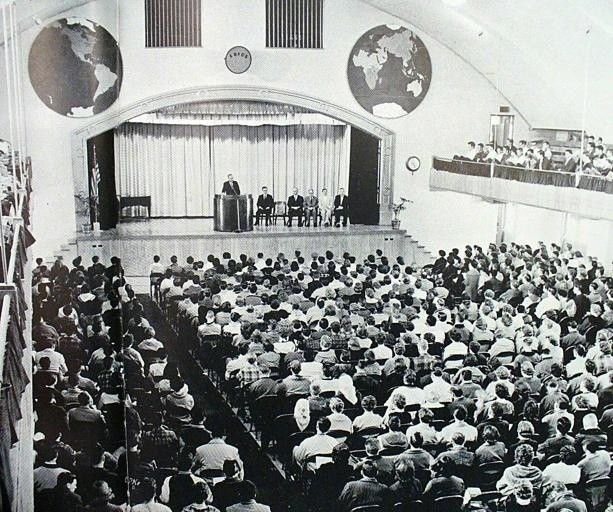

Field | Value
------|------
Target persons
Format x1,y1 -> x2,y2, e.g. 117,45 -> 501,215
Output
254,187 -> 276,225
320,187 -> 334,226
31,257 -> 268,512
146,242 -> 612,512
288,187 -> 304,227
304,190 -> 319,228
334,187 -> 350,228
221,173 -> 241,195
453,132 -> 613,188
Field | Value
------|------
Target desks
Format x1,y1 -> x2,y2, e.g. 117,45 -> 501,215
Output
121,195 -> 149,221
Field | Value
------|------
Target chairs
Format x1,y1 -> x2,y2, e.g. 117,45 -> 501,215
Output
258,200 -> 347,226
32,250 -> 613,510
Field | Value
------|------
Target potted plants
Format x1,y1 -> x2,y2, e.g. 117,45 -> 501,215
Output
387,197 -> 414,230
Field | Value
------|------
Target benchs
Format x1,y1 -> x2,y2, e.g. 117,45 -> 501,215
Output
453,139 -> 612,193
